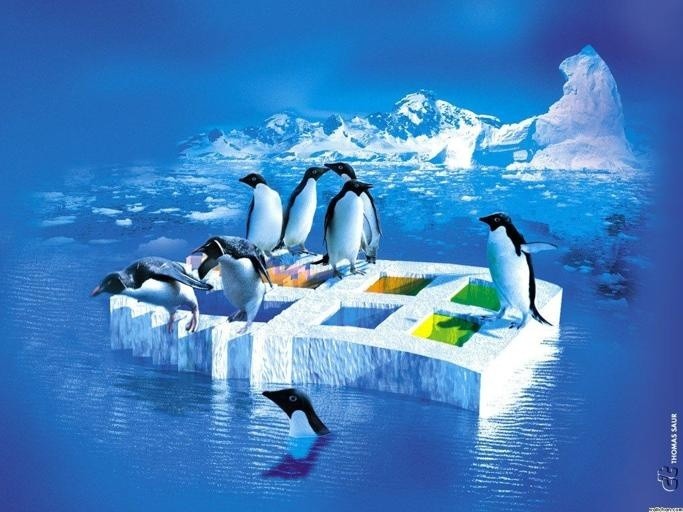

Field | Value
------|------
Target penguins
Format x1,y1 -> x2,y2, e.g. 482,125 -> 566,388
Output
89,256 -> 213,332
259,434 -> 327,481
190,235 -> 273,334
263,386 -> 332,435
323,179 -> 374,279
271,166 -> 332,256
238,172 -> 283,259
475,211 -> 559,333
324,162 -> 385,263
